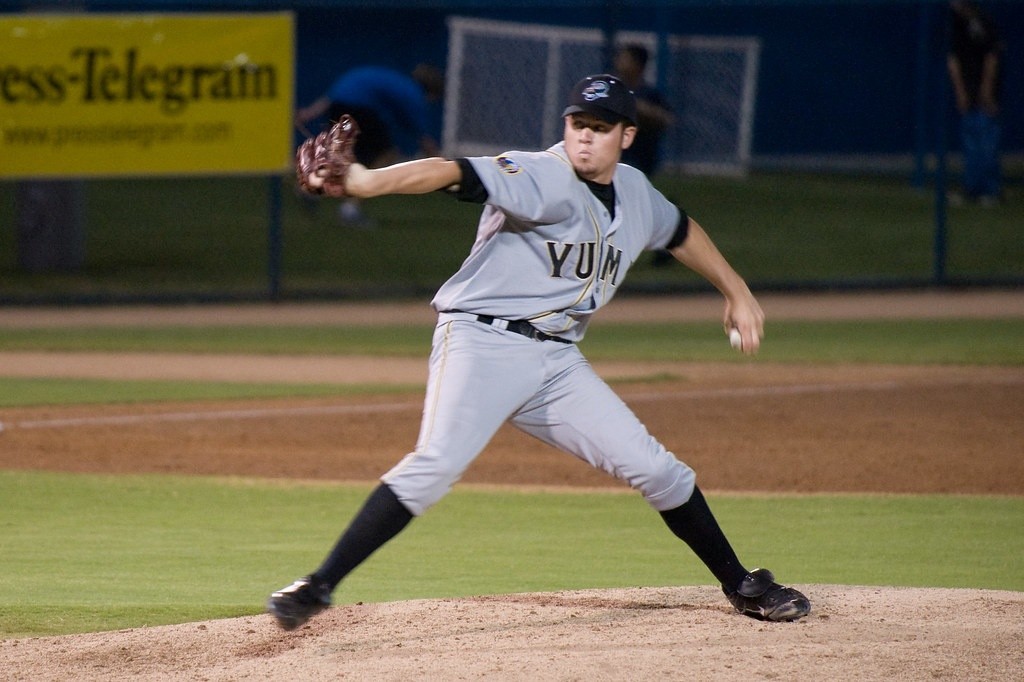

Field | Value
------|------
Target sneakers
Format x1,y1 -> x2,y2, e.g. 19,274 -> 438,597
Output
271,576 -> 331,630
721,568 -> 811,622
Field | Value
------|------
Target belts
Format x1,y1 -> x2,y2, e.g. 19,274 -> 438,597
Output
440,309 -> 572,345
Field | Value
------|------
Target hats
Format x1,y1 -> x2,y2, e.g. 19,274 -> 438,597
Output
562,74 -> 637,125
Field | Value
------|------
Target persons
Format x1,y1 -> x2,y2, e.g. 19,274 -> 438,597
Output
269,73 -> 810,630
296,60 -> 441,171
943,1 -> 1006,210
611,44 -> 675,177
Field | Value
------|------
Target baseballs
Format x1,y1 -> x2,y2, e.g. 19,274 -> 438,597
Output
729,323 -> 759,352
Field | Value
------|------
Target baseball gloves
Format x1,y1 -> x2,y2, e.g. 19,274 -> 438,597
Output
293,110 -> 360,201
